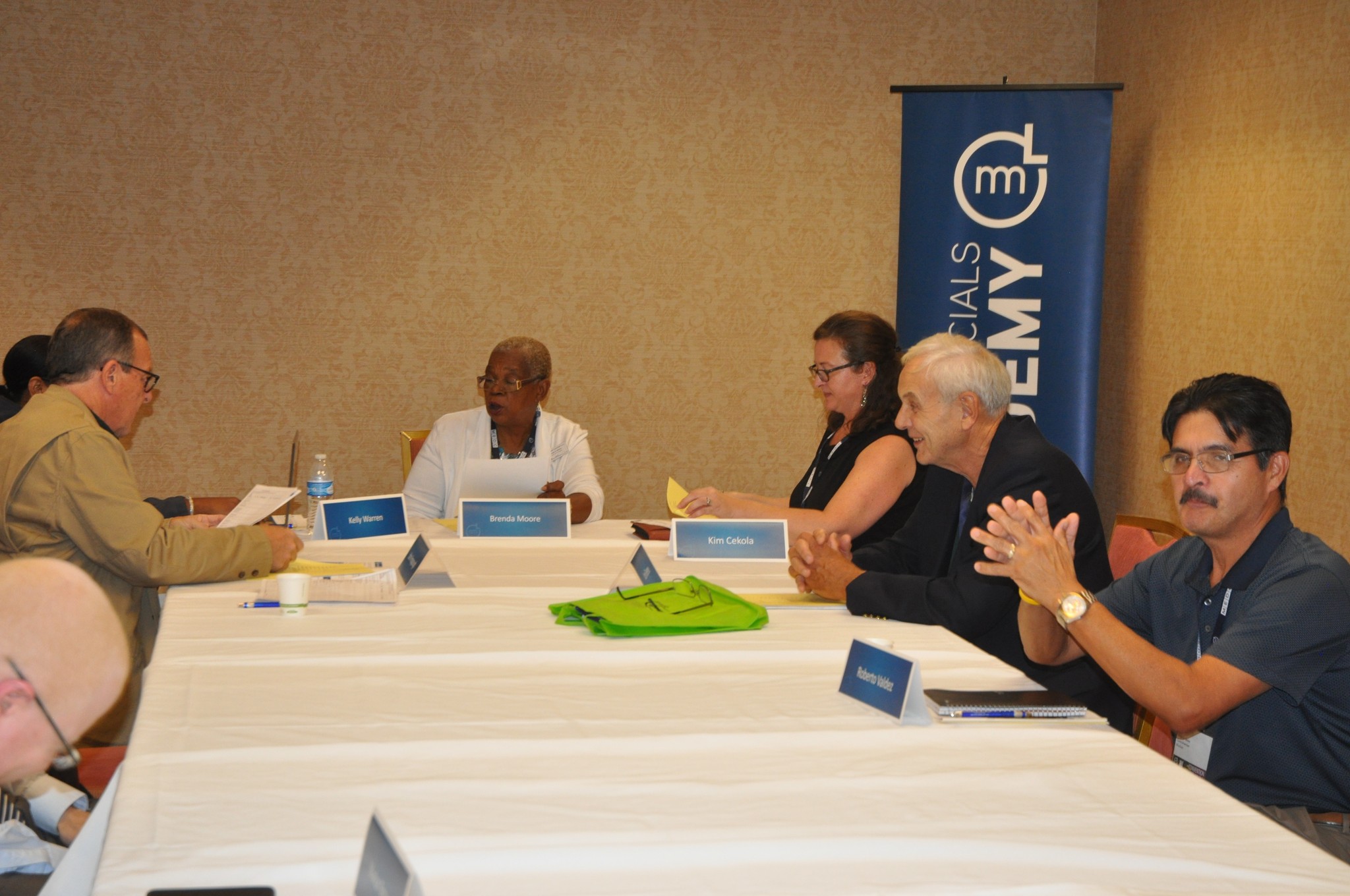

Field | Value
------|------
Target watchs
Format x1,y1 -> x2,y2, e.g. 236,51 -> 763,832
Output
1054,589 -> 1098,630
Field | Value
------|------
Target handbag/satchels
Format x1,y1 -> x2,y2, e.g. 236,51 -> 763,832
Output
548,577 -> 769,638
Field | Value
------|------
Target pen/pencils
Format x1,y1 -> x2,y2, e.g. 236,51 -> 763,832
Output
953,710 -> 1032,718
269,524 -> 296,528
238,601 -> 280,609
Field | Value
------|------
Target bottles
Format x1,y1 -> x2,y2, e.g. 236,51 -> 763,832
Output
307,453 -> 335,529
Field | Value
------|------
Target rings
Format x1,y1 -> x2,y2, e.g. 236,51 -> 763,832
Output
1007,543 -> 1016,559
721,490 -> 724,494
707,497 -> 710,505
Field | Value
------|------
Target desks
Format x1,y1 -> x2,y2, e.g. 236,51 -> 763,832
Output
88,517 -> 1350,896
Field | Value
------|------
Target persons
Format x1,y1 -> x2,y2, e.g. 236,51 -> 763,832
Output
0,307 -> 305,748
676,309 -> 938,553
787,332 -> 1134,737
0,334 -> 272,527
0,556 -> 134,896
399,336 -> 604,526
969,372 -> 1350,867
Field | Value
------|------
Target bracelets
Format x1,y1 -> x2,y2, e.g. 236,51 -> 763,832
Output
185,496 -> 194,515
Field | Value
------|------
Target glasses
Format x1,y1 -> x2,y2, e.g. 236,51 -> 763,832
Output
477,375 -> 544,391
100,360 -> 160,393
1,655 -> 80,772
808,359 -> 866,381
1157,448 -> 1269,475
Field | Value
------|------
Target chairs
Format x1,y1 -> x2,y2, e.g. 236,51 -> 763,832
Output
1103,517 -> 1200,763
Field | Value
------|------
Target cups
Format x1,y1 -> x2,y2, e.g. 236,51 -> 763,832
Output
290,529 -> 313,560
276,572 -> 310,612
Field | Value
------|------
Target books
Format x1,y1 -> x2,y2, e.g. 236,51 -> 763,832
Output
923,688 -> 1111,727
